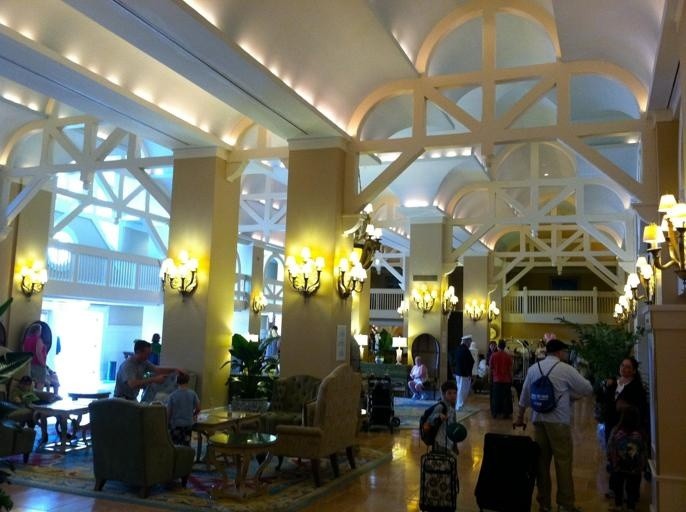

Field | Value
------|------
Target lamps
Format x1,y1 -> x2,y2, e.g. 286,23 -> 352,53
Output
160,251 -> 198,296
285,204 -> 382,300
16,260 -> 48,297
612,193 -> 686,327
397,284 -> 499,322
392,336 -> 407,366
253,291 -> 268,314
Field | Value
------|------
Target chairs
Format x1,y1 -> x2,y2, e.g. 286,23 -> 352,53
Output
412,333 -> 440,400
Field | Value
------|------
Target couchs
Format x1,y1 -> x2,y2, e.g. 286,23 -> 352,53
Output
360,363 -> 409,397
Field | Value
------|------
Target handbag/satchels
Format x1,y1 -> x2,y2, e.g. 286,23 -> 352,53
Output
607,431 -> 647,474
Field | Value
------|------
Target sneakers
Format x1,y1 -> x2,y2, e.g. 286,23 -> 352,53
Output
412,394 -> 428,400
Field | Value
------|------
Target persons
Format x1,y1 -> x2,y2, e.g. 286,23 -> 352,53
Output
148,334 -> 162,366
12,323 -> 52,415
420,382 -> 459,458
453,335 -> 548,419
166,374 -> 201,448
595,357 -> 651,512
258,326 -> 281,358
407,356 -> 428,400
114,341 -> 187,401
515,339 -> 594,512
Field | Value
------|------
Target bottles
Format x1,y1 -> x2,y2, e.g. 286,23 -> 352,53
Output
227,404 -> 232,419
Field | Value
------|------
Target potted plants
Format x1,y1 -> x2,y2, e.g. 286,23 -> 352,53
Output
375,351 -> 384,364
221,334 -> 280,430
558,318 -> 645,454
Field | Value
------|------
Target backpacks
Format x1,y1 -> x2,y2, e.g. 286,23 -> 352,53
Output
530,361 -> 562,413
420,402 -> 446,445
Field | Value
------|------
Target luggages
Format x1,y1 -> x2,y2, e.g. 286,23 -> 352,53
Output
475,423 -> 539,512
420,451 -> 459,512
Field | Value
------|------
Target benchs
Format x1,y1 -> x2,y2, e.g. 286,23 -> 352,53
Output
470,376 -> 488,394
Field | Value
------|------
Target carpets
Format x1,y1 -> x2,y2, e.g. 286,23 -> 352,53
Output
1,443 -> 392,512
394,395 -> 481,430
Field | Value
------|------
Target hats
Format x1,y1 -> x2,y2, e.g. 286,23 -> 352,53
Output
447,423 -> 466,442
546,340 -> 570,352
